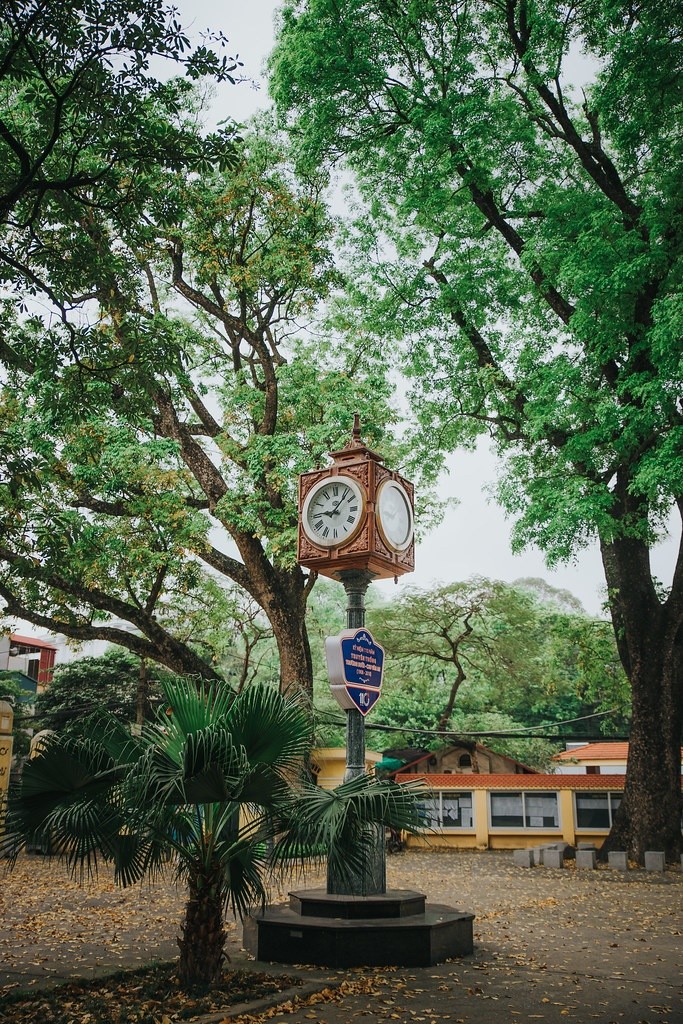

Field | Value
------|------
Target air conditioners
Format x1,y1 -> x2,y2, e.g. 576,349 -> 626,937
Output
444,769 -> 456,775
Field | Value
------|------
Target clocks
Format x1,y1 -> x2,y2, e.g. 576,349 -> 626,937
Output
303,475 -> 366,548
376,478 -> 414,552
